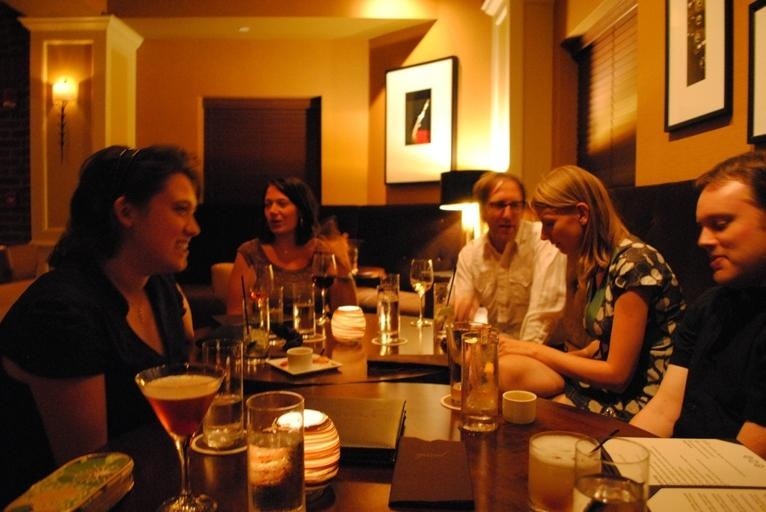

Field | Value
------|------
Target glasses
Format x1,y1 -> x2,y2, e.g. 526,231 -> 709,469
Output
486,201 -> 526,209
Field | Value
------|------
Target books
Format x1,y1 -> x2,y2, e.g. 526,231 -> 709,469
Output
303,394 -> 411,465
592,434 -> 766,512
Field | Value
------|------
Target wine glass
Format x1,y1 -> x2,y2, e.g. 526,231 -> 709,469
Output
248,263 -> 274,308
135,362 -> 227,512
409,258 -> 434,329
310,252 -> 338,327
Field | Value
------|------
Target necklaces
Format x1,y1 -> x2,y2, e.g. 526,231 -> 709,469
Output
120,287 -> 152,326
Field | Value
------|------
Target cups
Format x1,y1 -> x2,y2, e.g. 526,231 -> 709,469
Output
526,428 -> 651,512
244,390 -> 308,512
286,346 -> 314,373
200,337 -> 244,448
375,272 -> 400,344
241,297 -> 271,363
432,283 -> 456,341
268,285 -> 284,330
447,320 -> 537,434
291,281 -> 315,340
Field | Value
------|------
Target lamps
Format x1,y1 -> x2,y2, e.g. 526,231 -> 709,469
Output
52,76 -> 78,161
439,171 -> 491,234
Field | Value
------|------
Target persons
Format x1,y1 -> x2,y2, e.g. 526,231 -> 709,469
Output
446,172 -> 571,360
222,179 -> 360,316
630,152 -> 764,461
475,165 -> 690,428
0,148 -> 200,472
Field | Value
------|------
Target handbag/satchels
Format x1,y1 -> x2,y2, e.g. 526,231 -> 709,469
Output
2,454 -> 134,511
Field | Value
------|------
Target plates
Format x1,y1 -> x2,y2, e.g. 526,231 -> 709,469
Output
266,352 -> 342,376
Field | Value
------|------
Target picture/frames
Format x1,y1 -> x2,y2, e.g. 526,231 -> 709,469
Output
384,55 -> 459,187
748,1 -> 766,145
664,1 -> 733,132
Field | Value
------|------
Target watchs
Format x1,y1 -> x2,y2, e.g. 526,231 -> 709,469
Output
335,272 -> 355,284
